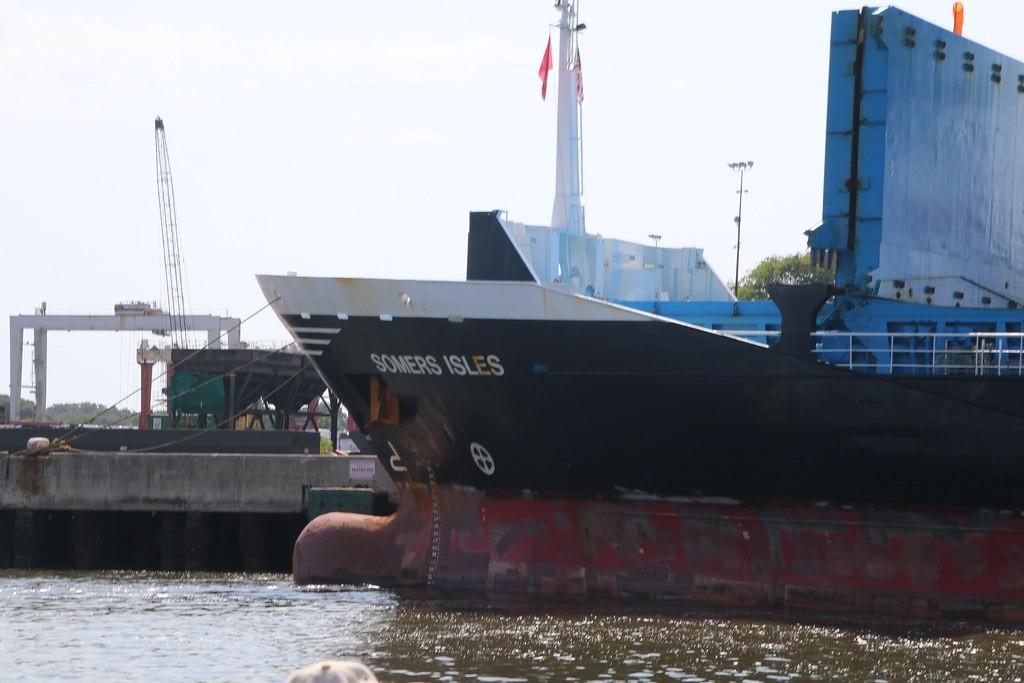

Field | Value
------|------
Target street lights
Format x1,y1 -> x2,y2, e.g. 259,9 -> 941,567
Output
727,160 -> 754,297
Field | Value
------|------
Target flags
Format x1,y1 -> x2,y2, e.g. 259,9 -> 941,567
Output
572,45 -> 584,106
537,34 -> 553,100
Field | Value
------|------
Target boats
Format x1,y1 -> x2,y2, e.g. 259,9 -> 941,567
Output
252,0 -> 1024,620
